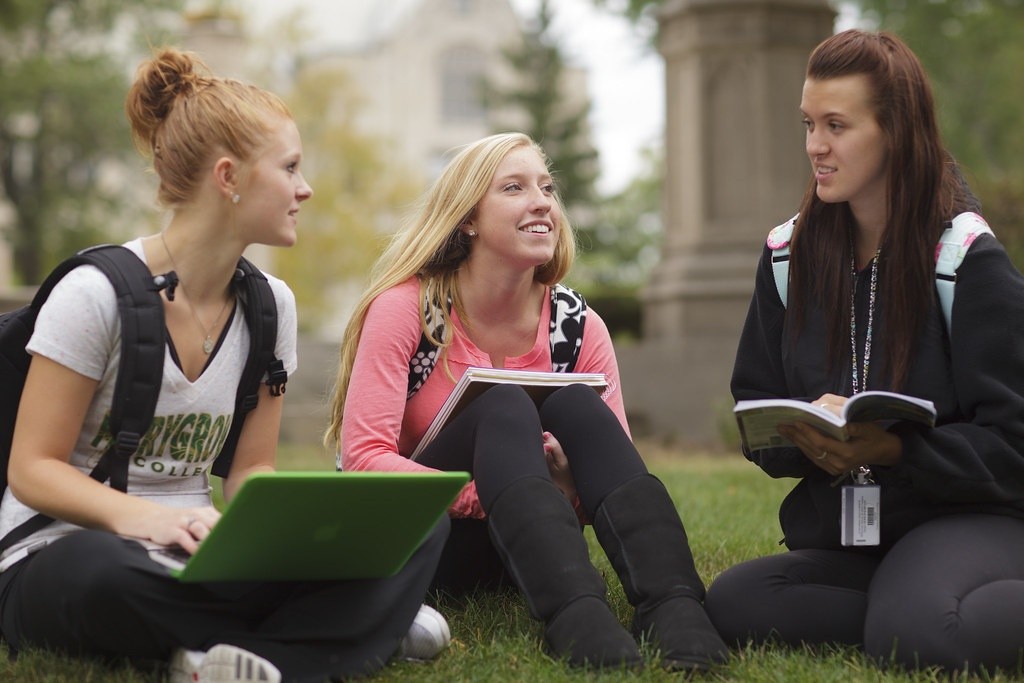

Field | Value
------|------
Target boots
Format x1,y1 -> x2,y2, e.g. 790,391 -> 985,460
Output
487,475 -> 643,671
591,472 -> 730,672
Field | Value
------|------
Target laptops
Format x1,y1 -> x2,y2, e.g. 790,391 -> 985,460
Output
115,471 -> 472,584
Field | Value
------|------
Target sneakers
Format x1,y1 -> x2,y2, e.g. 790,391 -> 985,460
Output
160,643 -> 283,683
394,604 -> 451,660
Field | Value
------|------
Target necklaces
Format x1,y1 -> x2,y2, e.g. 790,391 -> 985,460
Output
158,231 -> 231,356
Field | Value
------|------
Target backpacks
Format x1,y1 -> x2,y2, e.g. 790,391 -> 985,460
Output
0,243 -> 288,553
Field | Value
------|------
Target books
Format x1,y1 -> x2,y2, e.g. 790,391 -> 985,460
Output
733,390 -> 936,457
409,366 -> 607,467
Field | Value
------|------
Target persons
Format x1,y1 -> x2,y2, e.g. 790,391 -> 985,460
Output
706,27 -> 1024,682
326,130 -> 738,672
0,48 -> 449,682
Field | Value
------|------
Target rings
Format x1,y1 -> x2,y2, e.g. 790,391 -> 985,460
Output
817,451 -> 828,459
